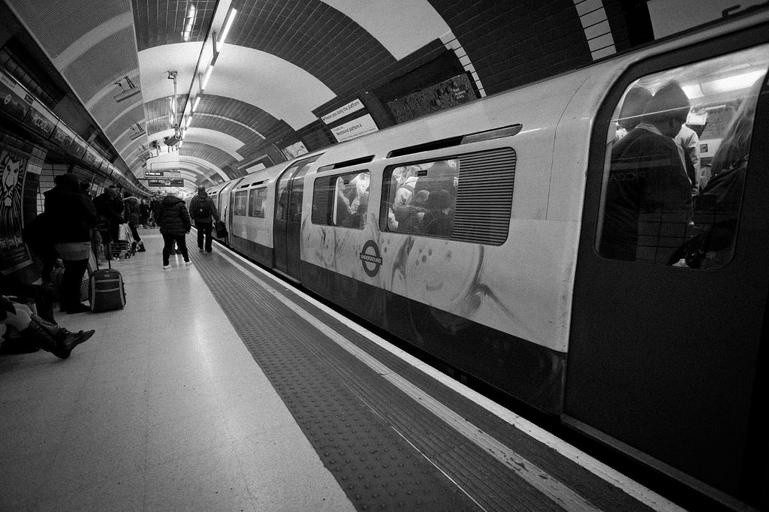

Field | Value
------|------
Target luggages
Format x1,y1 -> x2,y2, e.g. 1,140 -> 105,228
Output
88,242 -> 126,313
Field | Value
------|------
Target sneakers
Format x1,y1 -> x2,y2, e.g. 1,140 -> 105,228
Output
59,304 -> 91,314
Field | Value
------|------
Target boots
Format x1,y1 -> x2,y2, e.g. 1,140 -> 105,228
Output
17,313 -> 95,358
131,242 -> 146,252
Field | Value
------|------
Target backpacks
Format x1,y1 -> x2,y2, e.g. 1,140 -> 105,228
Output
195,197 -> 211,218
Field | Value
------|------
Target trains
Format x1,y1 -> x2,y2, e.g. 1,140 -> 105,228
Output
185,2 -> 768,512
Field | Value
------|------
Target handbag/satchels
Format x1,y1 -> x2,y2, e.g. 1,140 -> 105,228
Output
112,239 -> 128,257
215,220 -> 228,239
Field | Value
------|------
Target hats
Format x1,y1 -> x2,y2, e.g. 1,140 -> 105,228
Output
55,174 -> 80,186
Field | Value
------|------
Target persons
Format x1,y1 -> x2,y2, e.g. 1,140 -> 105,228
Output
1,174 -> 146,359
333,161 -> 459,235
600,74 -> 765,268
140,184 -> 220,269
